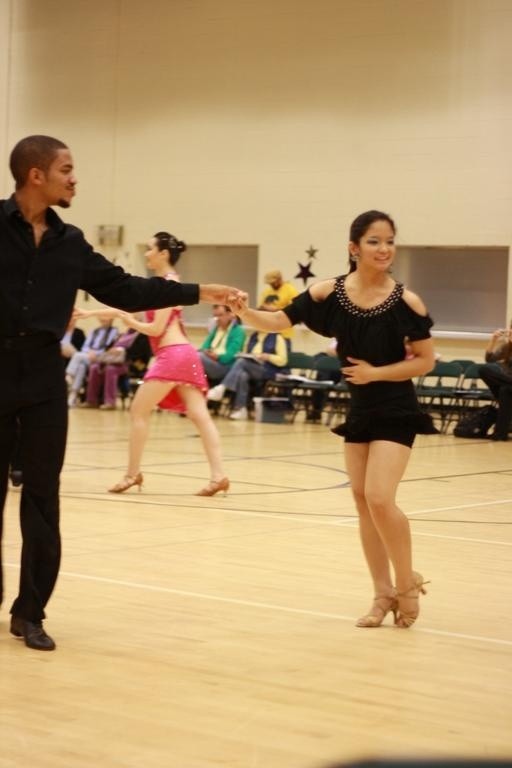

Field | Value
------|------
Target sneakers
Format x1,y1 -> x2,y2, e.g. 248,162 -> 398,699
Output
230,406 -> 248,419
206,384 -> 226,401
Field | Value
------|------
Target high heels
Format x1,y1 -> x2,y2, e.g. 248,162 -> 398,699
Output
108,473 -> 142,493
354,572 -> 428,628
195,477 -> 229,496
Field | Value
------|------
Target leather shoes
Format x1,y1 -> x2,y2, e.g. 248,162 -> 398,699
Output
8,470 -> 23,486
10,615 -> 55,650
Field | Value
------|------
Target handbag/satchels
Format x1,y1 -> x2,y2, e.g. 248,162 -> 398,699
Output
454,405 -> 499,436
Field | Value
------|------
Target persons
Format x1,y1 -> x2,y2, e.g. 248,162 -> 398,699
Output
221,208 -> 436,630
72,231 -> 233,500
197,294 -> 344,420
479,328 -> 512,441
257,270 -> 300,352
59,311 -> 153,409
0,135 -> 250,652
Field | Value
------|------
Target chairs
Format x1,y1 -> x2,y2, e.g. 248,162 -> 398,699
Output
269,351 -> 496,438
